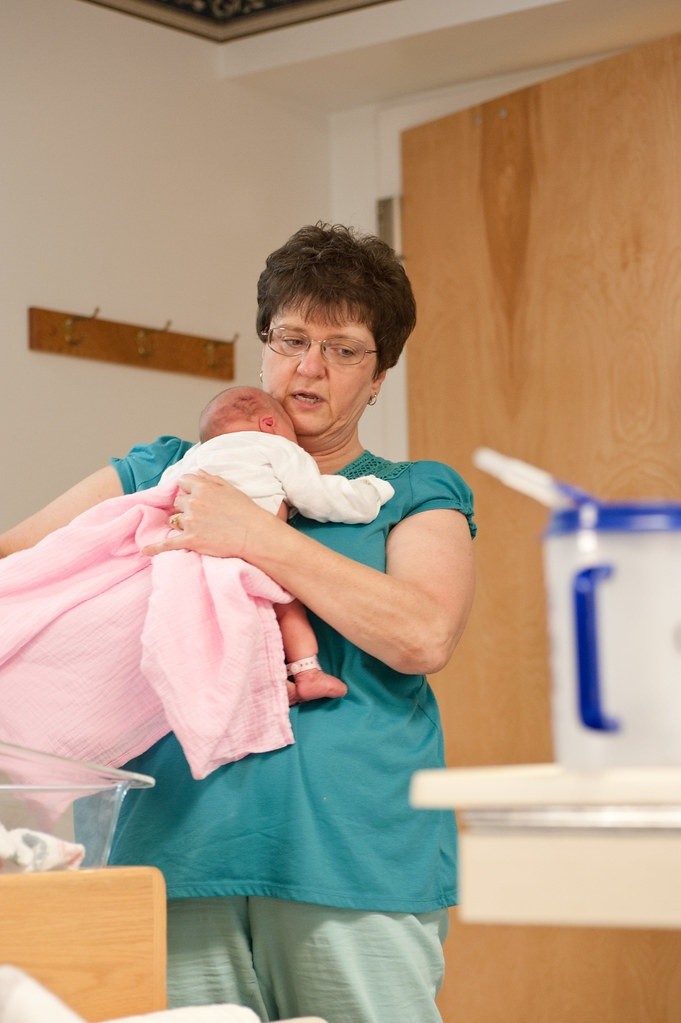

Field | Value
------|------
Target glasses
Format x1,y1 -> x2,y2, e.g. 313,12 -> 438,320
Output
259,325 -> 381,365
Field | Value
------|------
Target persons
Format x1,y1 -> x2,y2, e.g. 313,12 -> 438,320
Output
157,385 -> 396,706
0,223 -> 478,1023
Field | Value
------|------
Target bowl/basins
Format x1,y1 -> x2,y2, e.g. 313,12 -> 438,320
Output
0,740 -> 156,872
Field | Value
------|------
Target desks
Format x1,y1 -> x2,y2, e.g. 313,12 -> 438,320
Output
407,762 -> 681,932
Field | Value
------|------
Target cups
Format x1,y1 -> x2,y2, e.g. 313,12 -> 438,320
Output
540,499 -> 681,765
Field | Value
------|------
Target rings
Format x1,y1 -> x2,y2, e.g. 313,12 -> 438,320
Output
169,513 -> 182,530
